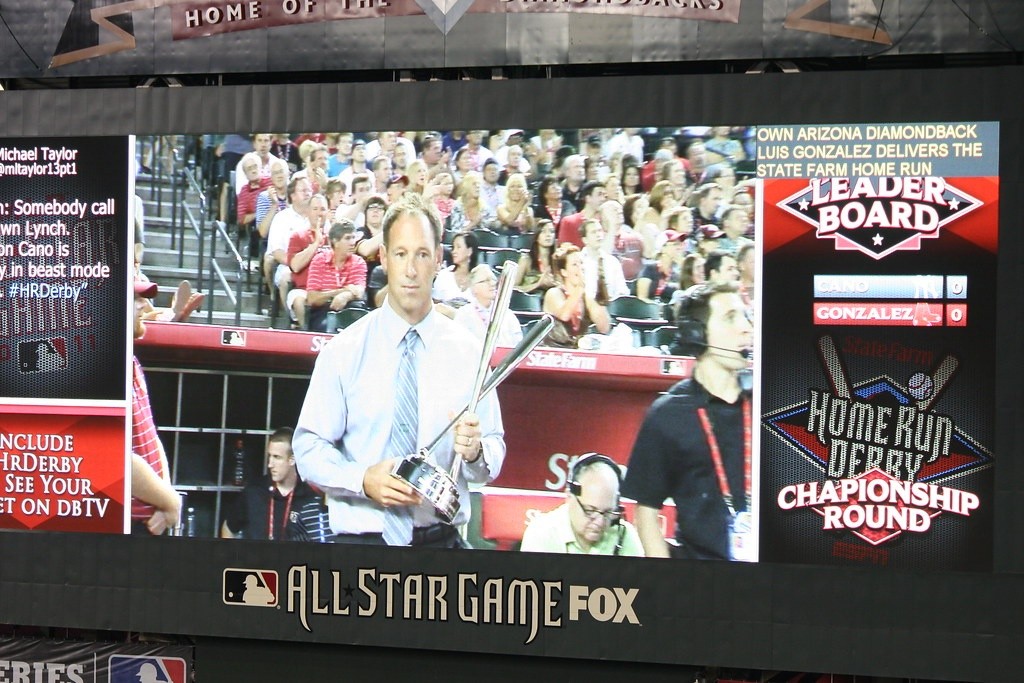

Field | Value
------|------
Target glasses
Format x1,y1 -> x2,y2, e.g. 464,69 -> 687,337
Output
572,494 -> 624,522
368,203 -> 385,210
479,276 -> 496,285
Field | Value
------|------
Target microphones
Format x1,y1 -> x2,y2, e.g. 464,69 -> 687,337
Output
694,341 -> 749,358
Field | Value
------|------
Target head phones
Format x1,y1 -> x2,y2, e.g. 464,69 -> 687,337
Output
570,453 -> 625,527
676,285 -> 714,356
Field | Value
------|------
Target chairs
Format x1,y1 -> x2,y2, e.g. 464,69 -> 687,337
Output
133,127 -> 755,354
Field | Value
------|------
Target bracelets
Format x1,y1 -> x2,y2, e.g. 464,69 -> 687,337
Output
462,441 -> 483,463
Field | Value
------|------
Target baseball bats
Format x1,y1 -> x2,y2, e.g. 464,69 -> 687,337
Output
449,259 -> 520,482
421,314 -> 554,458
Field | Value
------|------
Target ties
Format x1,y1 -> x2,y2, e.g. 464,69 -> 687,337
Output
596,256 -> 608,304
382,327 -> 421,546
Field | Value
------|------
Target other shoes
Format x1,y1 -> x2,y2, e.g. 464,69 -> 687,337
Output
250,259 -> 261,273
216,220 -> 227,238
180,293 -> 204,322
170,279 -> 191,322
142,163 -> 154,175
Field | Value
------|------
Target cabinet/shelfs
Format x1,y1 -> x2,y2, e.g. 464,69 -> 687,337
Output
140,367 -> 311,539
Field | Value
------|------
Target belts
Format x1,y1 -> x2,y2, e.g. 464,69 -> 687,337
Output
412,523 -> 456,544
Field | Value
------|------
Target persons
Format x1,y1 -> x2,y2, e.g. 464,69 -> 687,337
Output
130,193 -> 182,535
618,280 -> 753,558
520,452 -> 645,556
187,126 -> 755,349
221,426 -> 321,540
291,193 -> 505,548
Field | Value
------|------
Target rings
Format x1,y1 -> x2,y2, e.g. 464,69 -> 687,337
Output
467,437 -> 473,445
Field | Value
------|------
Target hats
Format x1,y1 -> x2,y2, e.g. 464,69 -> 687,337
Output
695,224 -> 727,240
134,280 -> 158,299
386,174 -> 409,187
588,132 -> 603,145
351,139 -> 366,149
484,157 -> 498,166
